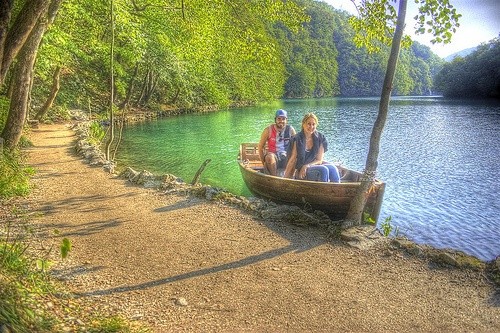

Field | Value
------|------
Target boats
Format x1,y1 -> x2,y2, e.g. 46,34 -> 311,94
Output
238,143 -> 386,226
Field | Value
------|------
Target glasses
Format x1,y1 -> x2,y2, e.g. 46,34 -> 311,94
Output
279,132 -> 283,142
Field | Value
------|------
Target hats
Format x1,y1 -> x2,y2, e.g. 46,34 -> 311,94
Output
276,109 -> 287,118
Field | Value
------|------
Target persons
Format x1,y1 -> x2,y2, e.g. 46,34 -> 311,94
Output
258,109 -> 297,179
283,112 -> 341,182
